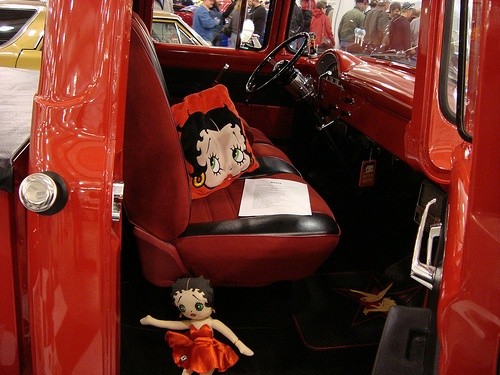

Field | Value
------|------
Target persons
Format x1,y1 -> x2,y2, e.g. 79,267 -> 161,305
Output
139,275 -> 254,375
230,0 -> 248,48
363,0 -> 432,55
209,0 -> 225,46
246,0 -> 266,47
192,0 -> 221,45
274,0 -> 334,50
338,0 -> 369,53
309,0 -> 335,54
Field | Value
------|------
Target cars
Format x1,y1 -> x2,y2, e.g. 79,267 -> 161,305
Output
1,0 -> 211,72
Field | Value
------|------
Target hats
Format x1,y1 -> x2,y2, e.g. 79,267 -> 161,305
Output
401,2 -> 415,11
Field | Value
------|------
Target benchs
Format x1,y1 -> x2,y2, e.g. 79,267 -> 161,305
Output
123,10 -> 341,286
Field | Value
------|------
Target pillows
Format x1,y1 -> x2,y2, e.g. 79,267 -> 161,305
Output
171,84 -> 259,199
